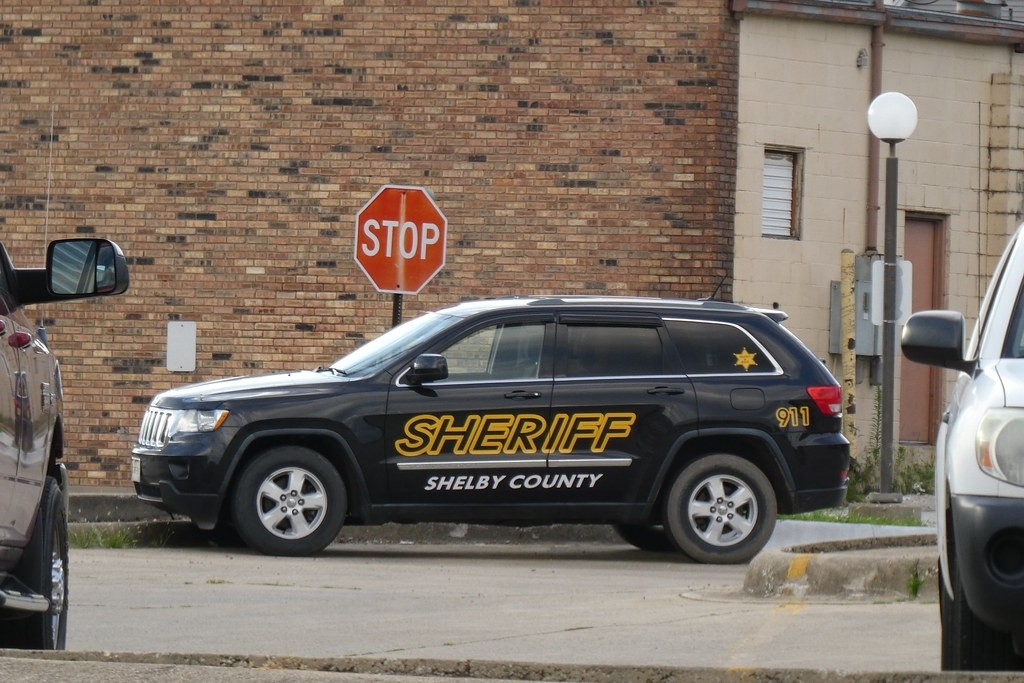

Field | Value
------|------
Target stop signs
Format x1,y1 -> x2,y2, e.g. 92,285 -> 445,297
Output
355,185 -> 448,296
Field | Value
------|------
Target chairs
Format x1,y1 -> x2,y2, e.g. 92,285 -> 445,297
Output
514,334 -> 541,378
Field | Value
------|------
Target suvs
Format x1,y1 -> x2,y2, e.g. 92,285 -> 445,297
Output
0,236 -> 132,652
128,293 -> 851,563
898,218 -> 1024,671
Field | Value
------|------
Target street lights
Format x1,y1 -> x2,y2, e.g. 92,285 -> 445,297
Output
864,90 -> 918,502
